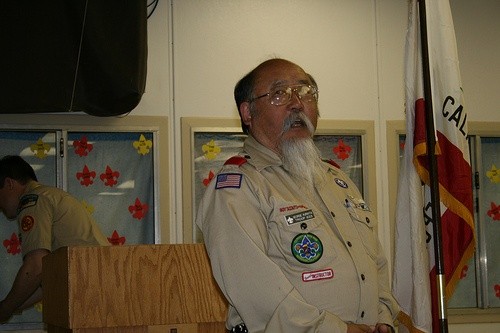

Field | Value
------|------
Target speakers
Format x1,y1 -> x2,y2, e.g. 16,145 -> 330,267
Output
0,0 -> 147,117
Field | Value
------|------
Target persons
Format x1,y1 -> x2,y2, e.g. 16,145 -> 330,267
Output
0,155 -> 113,322
196,59 -> 401,333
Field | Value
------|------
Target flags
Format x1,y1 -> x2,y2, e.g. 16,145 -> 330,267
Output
391,1 -> 477,333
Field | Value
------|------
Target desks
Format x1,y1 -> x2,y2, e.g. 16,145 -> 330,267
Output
41,243 -> 230,333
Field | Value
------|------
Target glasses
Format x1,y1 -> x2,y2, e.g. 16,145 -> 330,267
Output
249,85 -> 320,105
